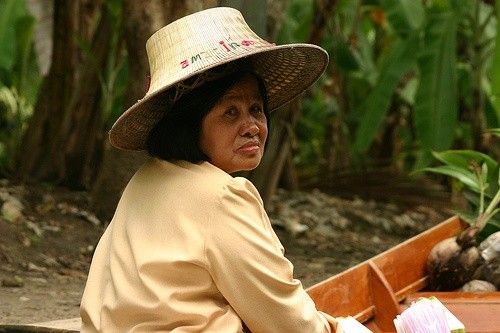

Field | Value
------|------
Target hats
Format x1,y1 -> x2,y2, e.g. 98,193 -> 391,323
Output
108,8 -> 330,152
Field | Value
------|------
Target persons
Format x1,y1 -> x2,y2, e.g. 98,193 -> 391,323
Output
80,7 -> 340,333
